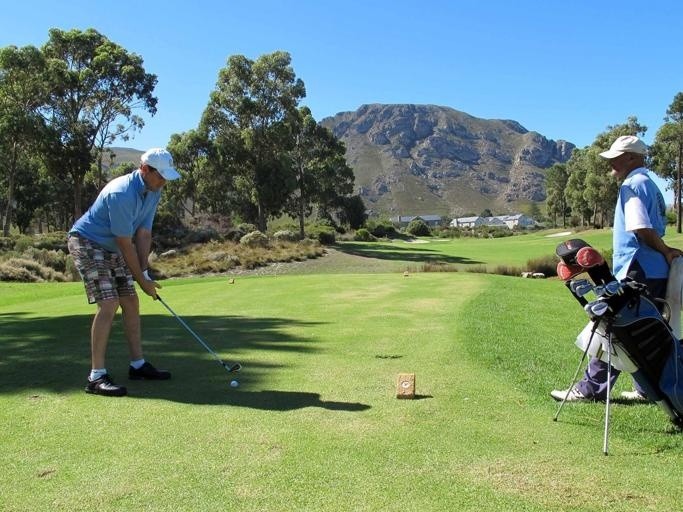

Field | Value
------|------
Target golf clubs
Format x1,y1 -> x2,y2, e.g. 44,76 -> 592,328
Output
555,239 -> 626,321
156,295 -> 242,373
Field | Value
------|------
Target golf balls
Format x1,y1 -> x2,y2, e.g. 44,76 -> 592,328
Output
231,381 -> 238,387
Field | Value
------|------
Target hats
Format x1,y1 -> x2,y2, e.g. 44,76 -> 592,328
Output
598,134 -> 647,159
141,147 -> 182,181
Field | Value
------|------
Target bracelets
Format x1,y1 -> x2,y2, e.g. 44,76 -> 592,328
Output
139,279 -> 145,286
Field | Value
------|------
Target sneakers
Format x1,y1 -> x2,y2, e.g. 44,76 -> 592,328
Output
550,386 -> 589,401
127,362 -> 171,381
621,389 -> 649,401
84,373 -> 127,396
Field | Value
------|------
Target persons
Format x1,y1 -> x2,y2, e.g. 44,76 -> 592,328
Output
66,147 -> 181,394
548,136 -> 683,401
